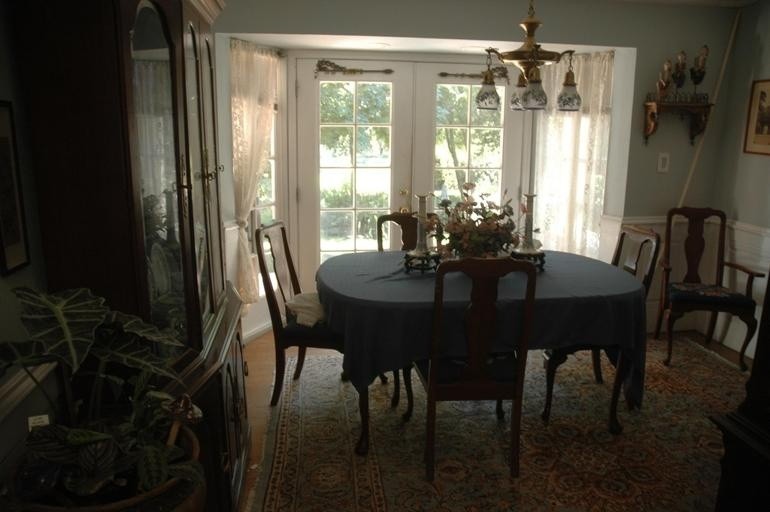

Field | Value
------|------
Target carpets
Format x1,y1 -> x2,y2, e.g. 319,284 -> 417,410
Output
239,333 -> 751,511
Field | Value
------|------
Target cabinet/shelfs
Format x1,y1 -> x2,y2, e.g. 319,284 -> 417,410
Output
164,307 -> 253,512
6,1 -> 239,404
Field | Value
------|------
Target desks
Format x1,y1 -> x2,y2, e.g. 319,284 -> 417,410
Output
316,249 -> 650,461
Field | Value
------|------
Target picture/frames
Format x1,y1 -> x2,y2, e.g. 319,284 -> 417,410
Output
742,79 -> 770,156
0,99 -> 34,280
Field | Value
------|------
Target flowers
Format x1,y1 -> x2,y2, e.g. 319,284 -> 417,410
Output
421,181 -> 541,258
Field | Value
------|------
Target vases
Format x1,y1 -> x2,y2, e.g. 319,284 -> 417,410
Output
459,249 -> 499,274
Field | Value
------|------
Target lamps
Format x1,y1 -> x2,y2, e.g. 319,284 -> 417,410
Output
475,0 -> 583,112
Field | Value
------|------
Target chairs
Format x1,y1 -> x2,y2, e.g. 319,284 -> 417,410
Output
652,207 -> 765,372
402,253 -> 535,487
378,210 -> 442,409
538,225 -> 662,422
254,219 -> 389,408
